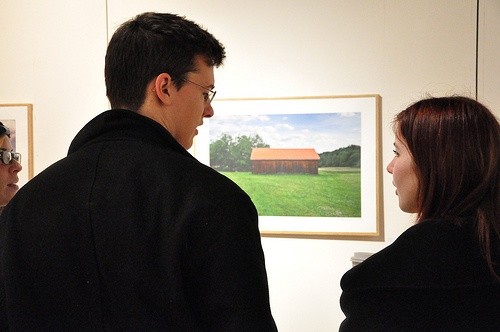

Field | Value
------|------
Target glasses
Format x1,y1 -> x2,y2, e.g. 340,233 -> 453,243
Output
183,78 -> 216,105
0,151 -> 22,164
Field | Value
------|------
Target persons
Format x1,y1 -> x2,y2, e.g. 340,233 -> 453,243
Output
339,96 -> 500,332
1,12 -> 277,332
0,122 -> 21,207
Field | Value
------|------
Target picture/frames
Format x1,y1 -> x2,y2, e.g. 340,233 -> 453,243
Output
193,93 -> 385,241
0,104 -> 34,187
